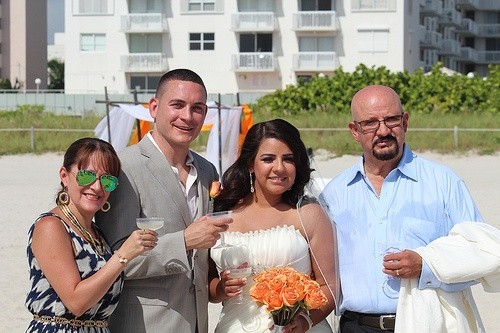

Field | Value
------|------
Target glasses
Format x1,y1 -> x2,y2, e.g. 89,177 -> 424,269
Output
354,112 -> 404,131
68,168 -> 119,192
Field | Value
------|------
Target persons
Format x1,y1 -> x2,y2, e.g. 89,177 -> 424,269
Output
95,67 -> 233,333
24,138 -> 158,333
208,120 -> 338,333
318,85 -> 483,333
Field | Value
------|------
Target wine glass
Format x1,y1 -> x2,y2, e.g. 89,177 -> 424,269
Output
205,210 -> 235,249
136,217 -> 165,256
224,247 -> 252,305
380,243 -> 402,293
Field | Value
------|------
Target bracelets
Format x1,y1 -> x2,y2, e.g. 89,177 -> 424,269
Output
114,249 -> 128,266
296,310 -> 313,333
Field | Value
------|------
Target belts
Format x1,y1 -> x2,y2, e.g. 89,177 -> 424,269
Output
343,309 -> 396,330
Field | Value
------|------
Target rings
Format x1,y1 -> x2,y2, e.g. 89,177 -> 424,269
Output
396,269 -> 399,276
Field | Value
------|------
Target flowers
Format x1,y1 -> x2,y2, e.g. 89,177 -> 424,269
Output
210,181 -> 221,202
249,266 -> 328,326
